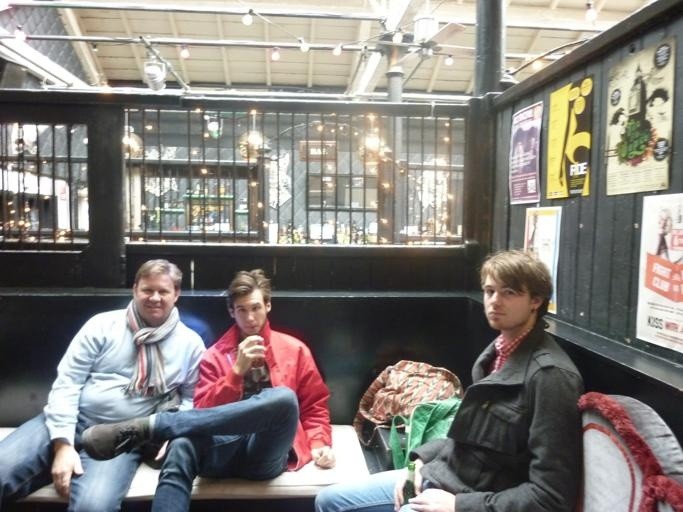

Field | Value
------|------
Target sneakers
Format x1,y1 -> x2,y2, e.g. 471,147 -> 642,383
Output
82,419 -> 149,460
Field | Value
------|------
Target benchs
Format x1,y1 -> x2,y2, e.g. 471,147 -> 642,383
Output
0,284 -> 683,512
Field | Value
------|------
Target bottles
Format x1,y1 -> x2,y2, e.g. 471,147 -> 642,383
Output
402,461 -> 416,503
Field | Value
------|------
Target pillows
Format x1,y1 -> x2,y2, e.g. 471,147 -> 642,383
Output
571,391 -> 683,512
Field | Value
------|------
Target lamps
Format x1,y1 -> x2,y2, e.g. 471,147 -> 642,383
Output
140,41 -> 169,92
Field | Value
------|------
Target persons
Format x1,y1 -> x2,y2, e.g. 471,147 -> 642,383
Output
0,257 -> 209,511
78,266 -> 334,511
310,249 -> 587,511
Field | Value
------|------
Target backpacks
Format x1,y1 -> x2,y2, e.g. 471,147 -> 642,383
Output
353,360 -> 463,469
